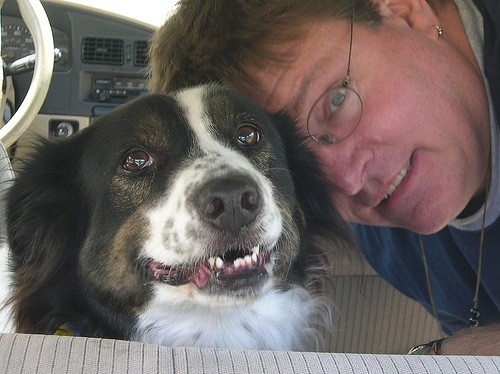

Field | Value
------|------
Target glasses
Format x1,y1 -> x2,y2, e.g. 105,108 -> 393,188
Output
281,16 -> 363,152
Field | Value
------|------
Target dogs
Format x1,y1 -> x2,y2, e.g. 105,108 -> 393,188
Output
0,81 -> 362,351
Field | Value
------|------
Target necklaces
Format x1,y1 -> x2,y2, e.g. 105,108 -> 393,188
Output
408,170 -> 488,333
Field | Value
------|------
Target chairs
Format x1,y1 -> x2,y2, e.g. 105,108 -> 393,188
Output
0,219 -> 500,373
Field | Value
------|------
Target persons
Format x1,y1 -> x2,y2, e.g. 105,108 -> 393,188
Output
144,0 -> 500,356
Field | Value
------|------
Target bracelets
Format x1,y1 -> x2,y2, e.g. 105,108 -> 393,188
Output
406,336 -> 448,355
